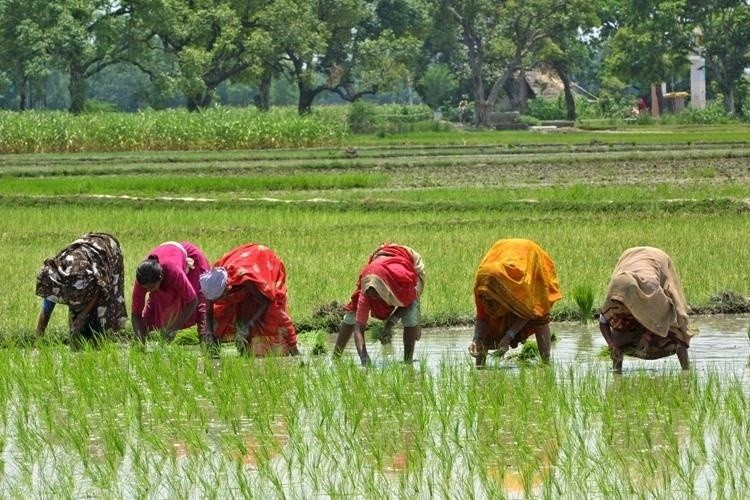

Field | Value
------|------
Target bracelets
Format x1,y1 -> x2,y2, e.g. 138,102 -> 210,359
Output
389,315 -> 399,325
250,320 -> 255,327
78,311 -> 89,321
642,333 -> 652,342
505,329 -> 515,341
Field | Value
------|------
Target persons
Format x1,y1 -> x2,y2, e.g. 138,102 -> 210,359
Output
470,239 -> 561,365
35,233 -> 126,345
131,241 -> 210,352
600,247 -> 689,372
200,243 -> 299,357
332,243 -> 425,368
459,95 -> 467,114
639,97 -> 646,111
